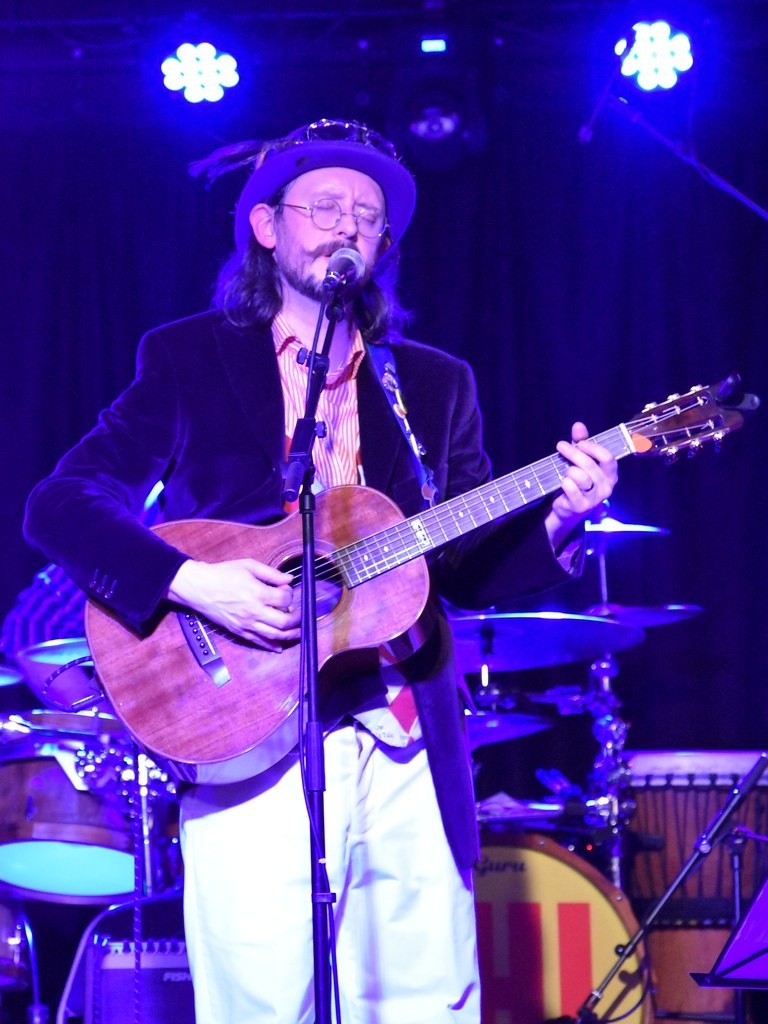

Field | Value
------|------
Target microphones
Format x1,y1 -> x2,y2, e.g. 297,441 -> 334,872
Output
322,247 -> 365,291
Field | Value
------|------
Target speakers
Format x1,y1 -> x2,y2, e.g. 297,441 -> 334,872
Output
83,933 -> 197,1024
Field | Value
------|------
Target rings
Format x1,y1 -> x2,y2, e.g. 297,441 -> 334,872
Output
580,481 -> 595,492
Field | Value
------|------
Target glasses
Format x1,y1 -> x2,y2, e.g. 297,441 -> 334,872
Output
257,119 -> 396,165
273,199 -> 390,239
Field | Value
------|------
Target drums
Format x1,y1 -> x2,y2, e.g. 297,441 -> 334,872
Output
0,665 -> 22,687
0,709 -> 183,907
593,751 -> 768,1024
476,830 -> 652,1024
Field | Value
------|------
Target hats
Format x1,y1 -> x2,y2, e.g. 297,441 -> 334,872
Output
234,119 -> 417,259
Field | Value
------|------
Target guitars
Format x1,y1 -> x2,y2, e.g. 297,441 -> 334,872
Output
83,370 -> 761,784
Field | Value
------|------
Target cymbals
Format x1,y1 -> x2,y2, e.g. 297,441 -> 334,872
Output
22,635 -> 95,667
584,517 -> 662,534
448,614 -> 645,675
581,605 -> 705,628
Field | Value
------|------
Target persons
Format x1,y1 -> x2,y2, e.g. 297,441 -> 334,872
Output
22,119 -> 619,1024
2,506 -> 169,715
